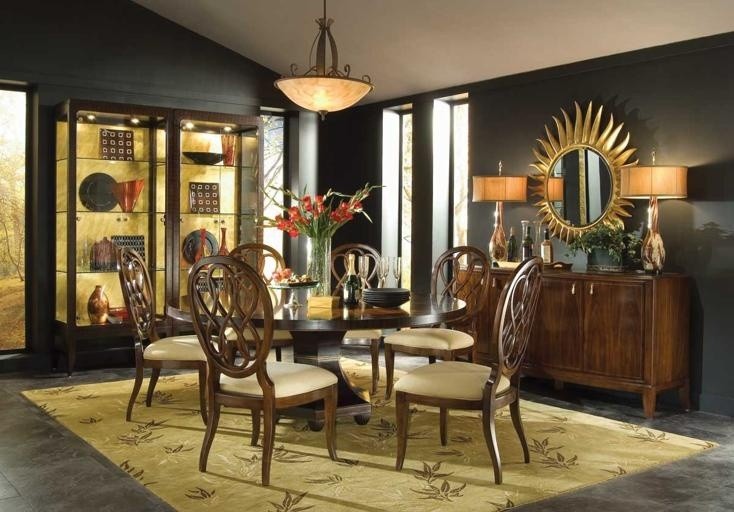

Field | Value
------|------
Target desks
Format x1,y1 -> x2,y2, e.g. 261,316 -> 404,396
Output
163,289 -> 468,431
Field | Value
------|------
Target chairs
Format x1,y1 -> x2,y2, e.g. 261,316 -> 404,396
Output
383,246 -> 491,402
392,258 -> 544,485
216,242 -> 296,363
332,243 -> 381,395
187,256 -> 338,488
116,247 -> 222,426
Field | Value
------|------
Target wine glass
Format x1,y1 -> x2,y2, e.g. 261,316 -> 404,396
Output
358,256 -> 368,288
374,256 -> 400,287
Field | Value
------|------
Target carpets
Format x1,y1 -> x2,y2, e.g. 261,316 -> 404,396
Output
20,354 -> 720,511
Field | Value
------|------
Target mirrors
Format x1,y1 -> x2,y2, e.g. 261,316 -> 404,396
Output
529,101 -> 639,247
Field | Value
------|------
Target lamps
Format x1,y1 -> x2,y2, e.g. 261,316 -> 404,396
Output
272,1 -> 373,122
547,175 -> 565,201
620,151 -> 690,277
472,160 -> 528,263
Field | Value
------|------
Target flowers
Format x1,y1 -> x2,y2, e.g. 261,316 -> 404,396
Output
255,182 -> 385,298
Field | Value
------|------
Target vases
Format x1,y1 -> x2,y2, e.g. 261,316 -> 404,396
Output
306,239 -> 331,297
86,284 -> 110,326
217,227 -> 229,259
193,229 -> 209,264
220,134 -> 236,167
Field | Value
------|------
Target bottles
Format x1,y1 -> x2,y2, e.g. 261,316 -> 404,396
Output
532,219 -> 541,257
540,228 -> 553,264
506,227 -> 517,262
342,253 -> 361,308
520,226 -> 533,261
518,220 -> 529,257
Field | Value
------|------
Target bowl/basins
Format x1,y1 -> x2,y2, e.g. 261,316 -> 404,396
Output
182,152 -> 226,165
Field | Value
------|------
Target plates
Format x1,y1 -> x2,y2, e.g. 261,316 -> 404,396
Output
361,288 -> 409,308
289,279 -> 319,286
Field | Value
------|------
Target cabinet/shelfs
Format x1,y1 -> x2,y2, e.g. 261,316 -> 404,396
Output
449,267 -> 690,418
51,98 -> 263,376
